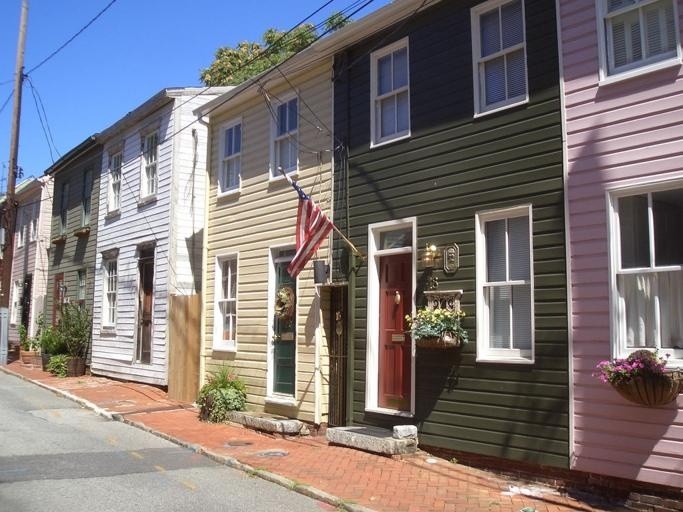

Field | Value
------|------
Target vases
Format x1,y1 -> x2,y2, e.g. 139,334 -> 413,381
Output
414,336 -> 463,350
611,370 -> 683,407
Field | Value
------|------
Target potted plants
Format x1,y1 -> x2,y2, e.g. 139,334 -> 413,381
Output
16,303 -> 92,376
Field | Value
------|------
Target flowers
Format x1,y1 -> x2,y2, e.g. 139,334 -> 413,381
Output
197,362 -> 247,422
591,348 -> 668,384
404,306 -> 468,334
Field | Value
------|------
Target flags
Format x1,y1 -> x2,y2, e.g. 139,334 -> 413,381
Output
284,173 -> 335,282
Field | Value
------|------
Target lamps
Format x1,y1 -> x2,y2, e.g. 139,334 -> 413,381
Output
420,241 -> 442,273
313,260 -> 330,285
